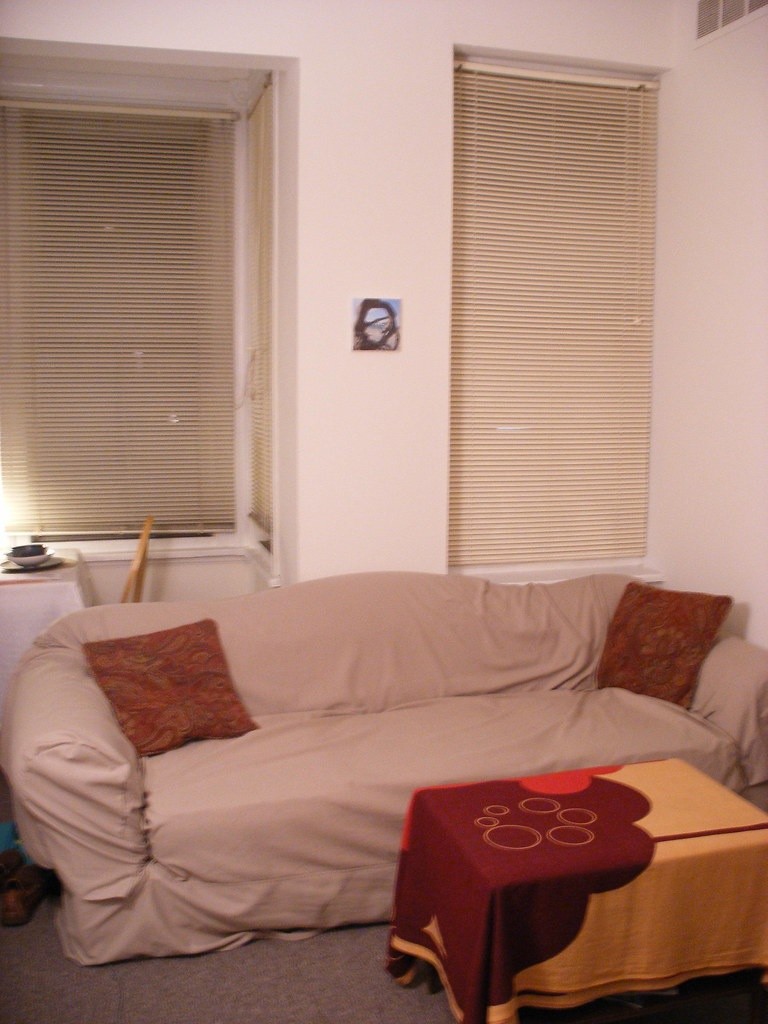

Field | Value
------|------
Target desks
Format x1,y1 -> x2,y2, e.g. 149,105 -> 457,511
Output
0,547 -> 98,716
388,758 -> 768,1024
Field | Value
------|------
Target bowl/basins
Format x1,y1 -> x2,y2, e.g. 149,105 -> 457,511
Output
6,551 -> 54,567
11,544 -> 44,556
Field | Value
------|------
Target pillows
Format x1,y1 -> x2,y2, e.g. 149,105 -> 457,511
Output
81,619 -> 259,755
597,581 -> 734,710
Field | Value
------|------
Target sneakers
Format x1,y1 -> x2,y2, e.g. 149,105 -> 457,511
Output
0,849 -> 23,881
0,865 -> 47,926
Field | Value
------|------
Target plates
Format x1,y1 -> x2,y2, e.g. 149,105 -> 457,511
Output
0,557 -> 64,572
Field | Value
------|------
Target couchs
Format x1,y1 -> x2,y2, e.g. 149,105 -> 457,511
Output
4,572 -> 768,970
